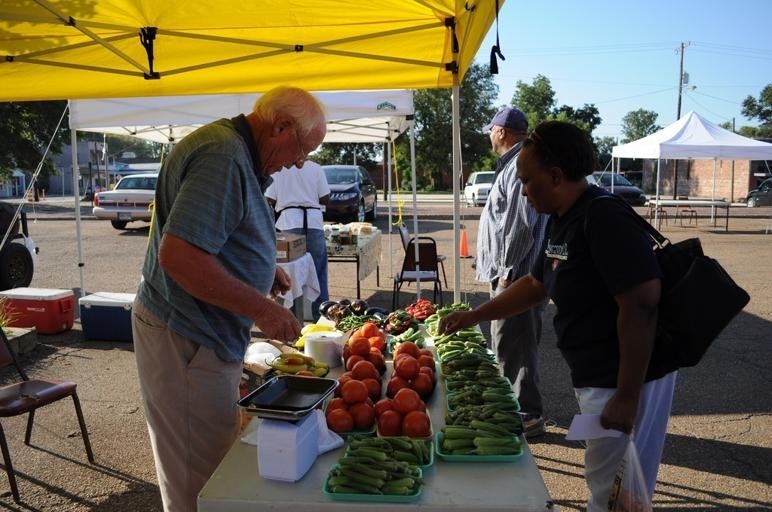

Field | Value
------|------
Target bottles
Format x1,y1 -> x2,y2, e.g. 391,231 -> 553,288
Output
482,108 -> 528,131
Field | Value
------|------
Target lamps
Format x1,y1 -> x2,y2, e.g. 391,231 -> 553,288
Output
672,194 -> 699,226
0,328 -> 96,507
395,221 -> 451,290
392,234 -> 443,309
644,196 -> 670,227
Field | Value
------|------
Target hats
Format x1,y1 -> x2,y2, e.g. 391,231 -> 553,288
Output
745,179 -> 772,207
583,171 -> 648,209
320,165 -> 381,223
93,173 -> 159,229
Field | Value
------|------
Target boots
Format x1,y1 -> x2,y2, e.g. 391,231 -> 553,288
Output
462,171 -> 496,206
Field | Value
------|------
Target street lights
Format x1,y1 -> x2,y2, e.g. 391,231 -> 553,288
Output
584,194 -> 750,367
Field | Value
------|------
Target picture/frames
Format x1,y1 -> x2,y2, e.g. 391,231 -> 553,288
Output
323,224 -> 383,300
198,314 -> 554,510
646,199 -> 731,234
265,251 -> 313,325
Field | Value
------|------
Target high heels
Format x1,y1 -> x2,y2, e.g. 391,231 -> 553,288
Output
460,230 -> 473,260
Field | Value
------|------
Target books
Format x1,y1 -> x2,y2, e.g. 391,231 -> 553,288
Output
272,234 -> 309,263
79,289 -> 137,338
239,331 -> 301,387
1,287 -> 78,338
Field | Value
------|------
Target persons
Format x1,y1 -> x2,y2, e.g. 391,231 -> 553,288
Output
437,121 -> 679,512
477,108 -> 549,437
265,160 -> 331,322
131,86 -> 326,512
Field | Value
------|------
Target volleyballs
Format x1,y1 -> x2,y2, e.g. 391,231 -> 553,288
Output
317,299 -> 388,322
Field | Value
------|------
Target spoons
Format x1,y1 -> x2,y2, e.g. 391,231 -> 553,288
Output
327,316 -> 384,431
375,339 -> 435,439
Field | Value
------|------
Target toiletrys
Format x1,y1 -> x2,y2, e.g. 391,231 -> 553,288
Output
264,324 -> 335,376
384,299 -> 435,335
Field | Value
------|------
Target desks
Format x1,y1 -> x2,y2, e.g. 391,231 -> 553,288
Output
674,83 -> 698,196
